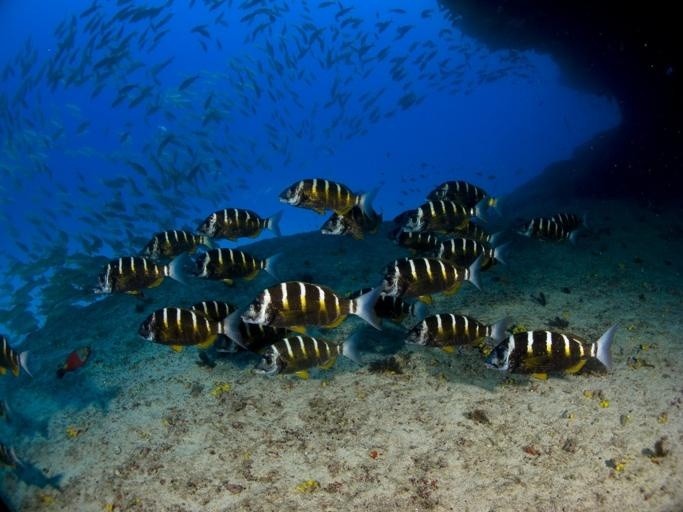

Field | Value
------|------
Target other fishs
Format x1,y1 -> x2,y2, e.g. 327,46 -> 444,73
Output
0,0 -> 537,333
